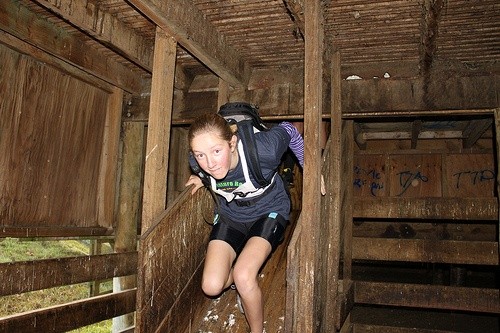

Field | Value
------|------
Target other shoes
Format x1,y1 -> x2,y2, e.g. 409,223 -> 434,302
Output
231,285 -> 245,313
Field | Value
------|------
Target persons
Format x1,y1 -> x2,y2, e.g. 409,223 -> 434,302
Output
185,111 -> 326,333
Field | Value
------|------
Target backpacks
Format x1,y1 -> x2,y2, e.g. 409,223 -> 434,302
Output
188,102 -> 294,214
